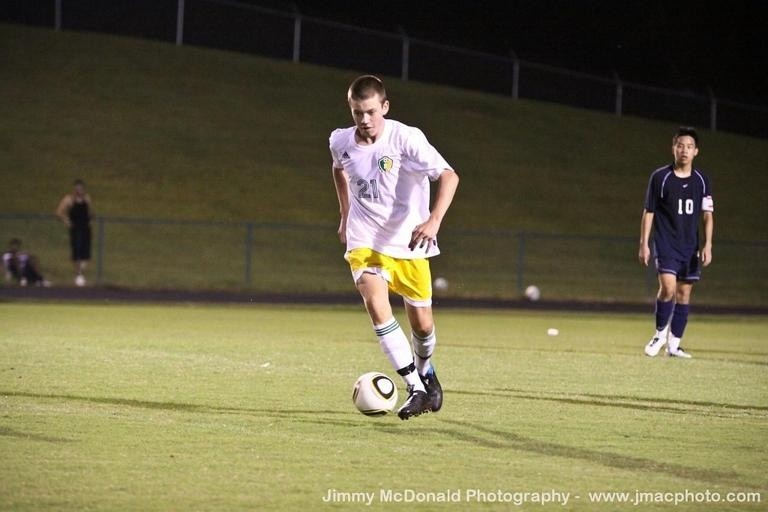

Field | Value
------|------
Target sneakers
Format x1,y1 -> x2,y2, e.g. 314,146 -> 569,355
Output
664,347 -> 692,360
644,331 -> 669,358
418,362 -> 444,413
398,385 -> 431,421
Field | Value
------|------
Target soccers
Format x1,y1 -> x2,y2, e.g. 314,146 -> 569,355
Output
353,372 -> 398,416
526,285 -> 540,300
435,278 -> 449,290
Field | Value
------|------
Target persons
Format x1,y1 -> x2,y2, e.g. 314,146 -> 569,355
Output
639,126 -> 714,359
329,75 -> 460,420
56,180 -> 94,287
3,238 -> 53,288
